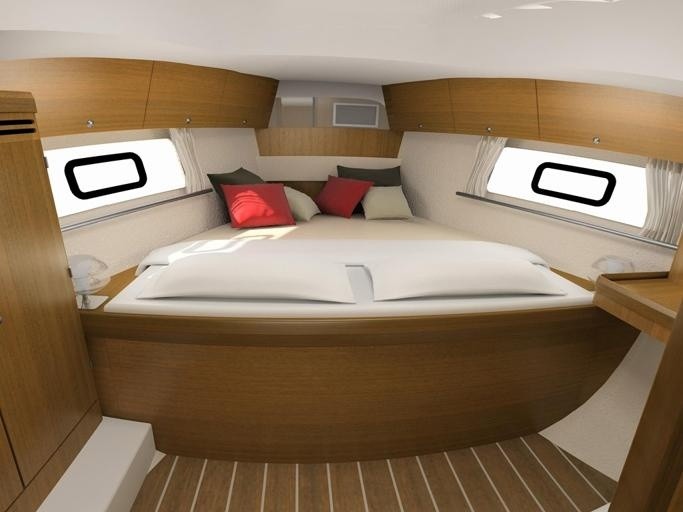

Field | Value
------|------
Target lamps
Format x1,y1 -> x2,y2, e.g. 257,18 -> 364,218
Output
67,254 -> 111,311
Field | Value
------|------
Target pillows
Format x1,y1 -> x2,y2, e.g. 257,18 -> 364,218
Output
137,243 -> 356,303
365,242 -> 567,302
204,164 -> 413,229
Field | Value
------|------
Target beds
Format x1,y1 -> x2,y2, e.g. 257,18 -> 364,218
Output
74,208 -> 641,461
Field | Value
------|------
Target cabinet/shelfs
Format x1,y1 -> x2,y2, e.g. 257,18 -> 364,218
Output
1,85 -> 102,512
